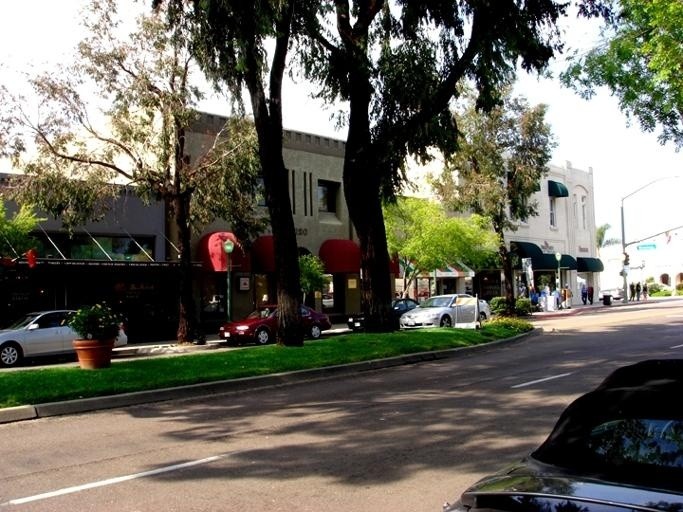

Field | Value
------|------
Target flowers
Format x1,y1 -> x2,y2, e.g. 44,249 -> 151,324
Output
61,300 -> 130,340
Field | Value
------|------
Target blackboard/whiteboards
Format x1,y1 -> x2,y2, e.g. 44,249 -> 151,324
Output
603,295 -> 614,305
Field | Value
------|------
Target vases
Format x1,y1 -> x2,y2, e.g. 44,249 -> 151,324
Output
72,339 -> 114,370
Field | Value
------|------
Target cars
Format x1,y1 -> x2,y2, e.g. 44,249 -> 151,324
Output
440,358 -> 683,512
218,304 -> 332,347
400,293 -> 489,332
347,298 -> 420,333
0,309 -> 128,366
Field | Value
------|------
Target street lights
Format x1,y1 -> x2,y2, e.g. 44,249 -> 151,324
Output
219,236 -> 234,322
555,252 -> 563,309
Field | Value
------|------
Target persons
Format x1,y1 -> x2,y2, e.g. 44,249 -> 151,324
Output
635,281 -> 642,301
518,281 -> 594,309
628,281 -> 635,302
642,282 -> 648,300
